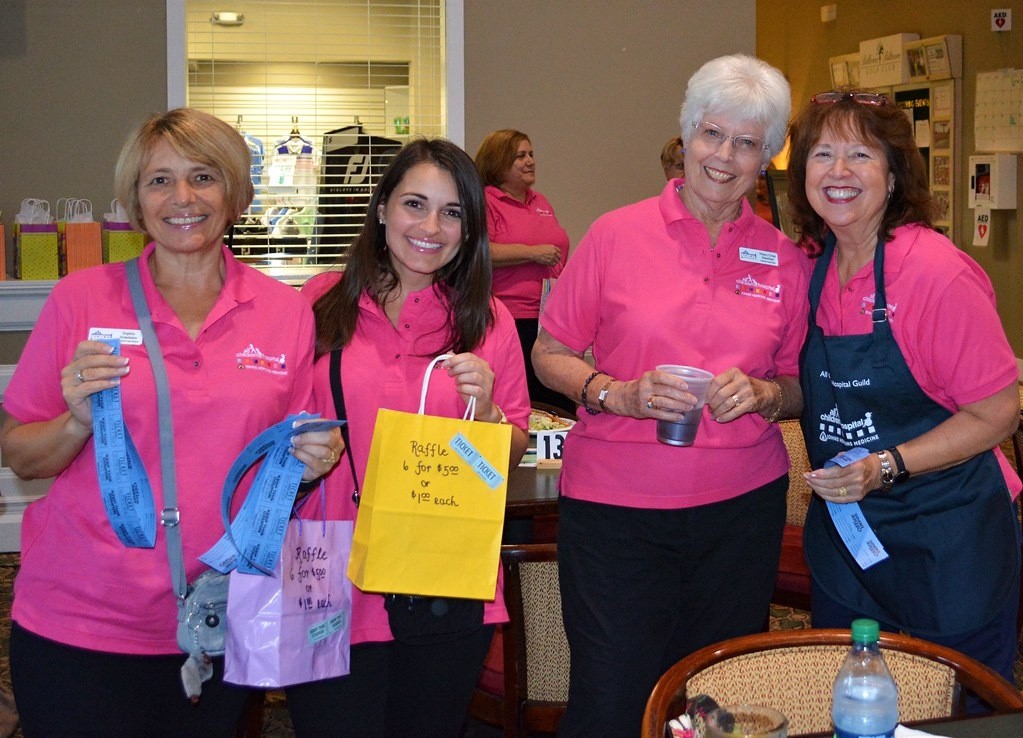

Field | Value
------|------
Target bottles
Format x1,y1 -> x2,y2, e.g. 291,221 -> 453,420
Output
831,619 -> 898,738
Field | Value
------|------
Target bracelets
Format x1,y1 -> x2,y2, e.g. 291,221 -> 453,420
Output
885,446 -> 910,484
765,377 -> 786,423
497,405 -> 508,424
582,371 -> 608,415
598,378 -> 618,414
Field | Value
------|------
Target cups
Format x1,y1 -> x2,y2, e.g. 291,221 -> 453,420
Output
656,365 -> 714,447
704,704 -> 788,738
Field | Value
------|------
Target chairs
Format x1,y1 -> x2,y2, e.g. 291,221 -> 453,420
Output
471,544 -> 572,738
641,627 -> 1023,738
762,419 -> 813,613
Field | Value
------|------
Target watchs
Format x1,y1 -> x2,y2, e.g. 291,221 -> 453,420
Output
874,450 -> 894,491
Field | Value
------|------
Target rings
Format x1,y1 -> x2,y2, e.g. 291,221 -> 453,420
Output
732,394 -> 740,407
838,487 -> 847,496
323,450 -> 335,465
76,370 -> 84,384
646,396 -> 654,410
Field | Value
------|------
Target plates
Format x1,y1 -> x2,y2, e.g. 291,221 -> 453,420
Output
528,419 -> 577,435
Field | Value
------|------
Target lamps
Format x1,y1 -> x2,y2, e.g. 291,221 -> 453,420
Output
210,11 -> 245,26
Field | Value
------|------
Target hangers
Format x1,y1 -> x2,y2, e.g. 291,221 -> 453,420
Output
234,114 -> 363,224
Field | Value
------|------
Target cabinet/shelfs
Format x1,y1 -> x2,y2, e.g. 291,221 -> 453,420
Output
0,277 -> 63,553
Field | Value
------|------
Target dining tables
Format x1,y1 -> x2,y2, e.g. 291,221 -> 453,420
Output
500,399 -> 578,542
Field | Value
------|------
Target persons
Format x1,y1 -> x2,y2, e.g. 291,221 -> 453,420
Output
660,134 -> 779,230
0,109 -> 344,738
300,138 -> 528,738
474,129 -> 569,387
531,54 -> 813,738
789,87 -> 1023,722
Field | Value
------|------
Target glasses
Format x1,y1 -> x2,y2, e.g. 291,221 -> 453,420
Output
806,91 -> 890,108
688,117 -> 772,158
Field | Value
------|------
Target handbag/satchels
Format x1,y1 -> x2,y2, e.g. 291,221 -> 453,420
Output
222,477 -> 355,689
173,569 -> 235,696
12,197 -> 144,282
346,354 -> 512,601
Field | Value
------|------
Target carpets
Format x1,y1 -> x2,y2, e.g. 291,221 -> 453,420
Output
1,548 -> 815,737
